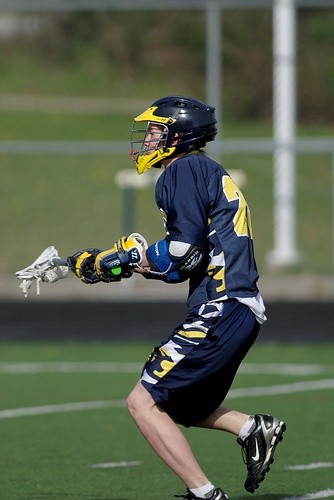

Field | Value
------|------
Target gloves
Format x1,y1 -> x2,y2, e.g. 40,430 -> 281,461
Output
67,247 -> 103,284
96,233 -> 148,283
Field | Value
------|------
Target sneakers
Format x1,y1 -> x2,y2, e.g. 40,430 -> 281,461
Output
236,413 -> 286,493
174,487 -> 230,500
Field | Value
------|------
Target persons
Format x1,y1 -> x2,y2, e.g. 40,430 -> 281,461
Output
67,96 -> 285,500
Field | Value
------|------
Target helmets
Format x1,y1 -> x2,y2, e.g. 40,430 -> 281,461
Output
130,96 -> 218,175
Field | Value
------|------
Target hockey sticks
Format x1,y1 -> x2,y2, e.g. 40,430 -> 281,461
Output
14,245 -> 140,284
